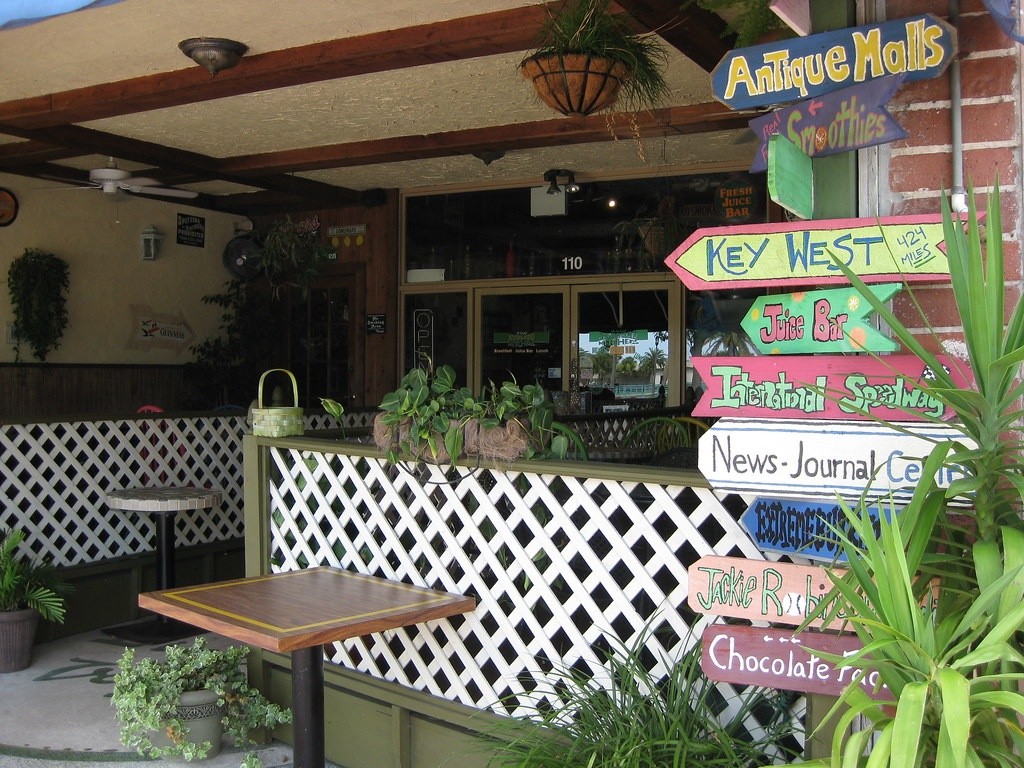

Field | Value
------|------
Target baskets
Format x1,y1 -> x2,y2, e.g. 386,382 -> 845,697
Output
251,368 -> 303,438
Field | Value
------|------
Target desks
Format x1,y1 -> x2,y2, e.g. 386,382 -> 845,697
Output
101,485 -> 224,644
138,566 -> 477,768
565,443 -> 652,465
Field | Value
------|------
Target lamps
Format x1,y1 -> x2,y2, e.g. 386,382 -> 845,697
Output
544,168 -> 579,194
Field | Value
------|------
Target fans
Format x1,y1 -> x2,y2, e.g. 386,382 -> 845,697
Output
28,156 -> 199,199
222,235 -> 266,282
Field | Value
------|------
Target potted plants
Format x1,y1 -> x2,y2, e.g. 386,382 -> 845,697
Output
513,0 -> 673,166
318,350 -> 570,469
0,527 -> 80,674
614,203 -> 693,275
108,634 -> 293,768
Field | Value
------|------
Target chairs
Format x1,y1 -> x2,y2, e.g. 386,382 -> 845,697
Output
550,417 -> 710,468
